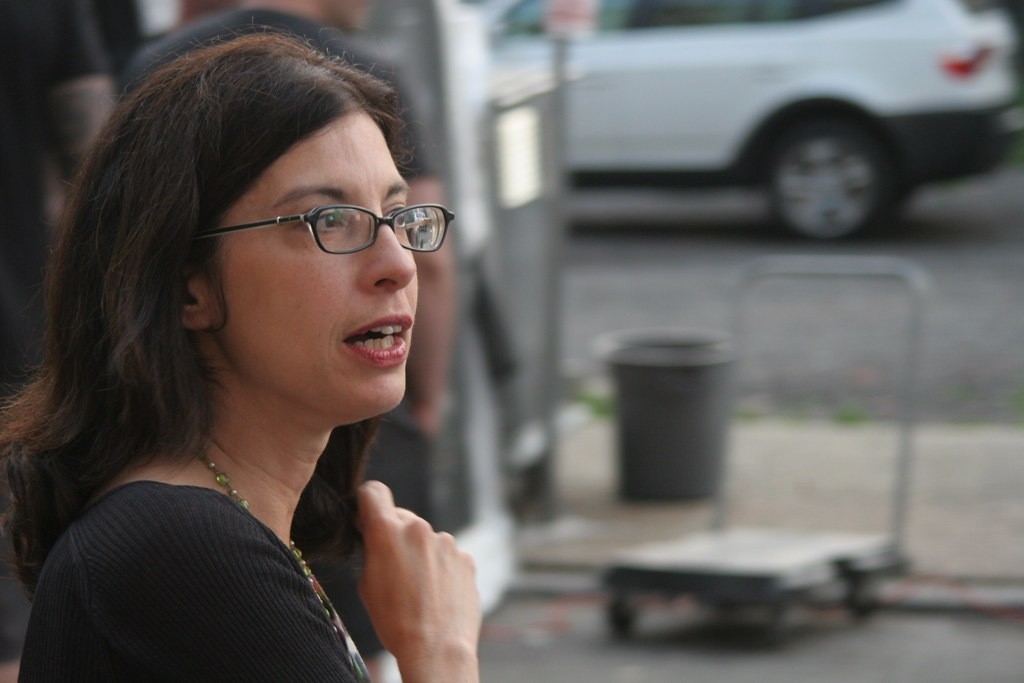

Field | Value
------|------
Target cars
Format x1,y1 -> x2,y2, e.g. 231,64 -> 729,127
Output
489,0 -> 1024,246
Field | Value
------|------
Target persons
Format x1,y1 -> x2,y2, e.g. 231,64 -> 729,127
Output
117,1 -> 458,683
1,0 -> 117,392
2,31 -> 484,682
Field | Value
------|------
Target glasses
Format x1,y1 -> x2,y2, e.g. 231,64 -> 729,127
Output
187,204 -> 456,253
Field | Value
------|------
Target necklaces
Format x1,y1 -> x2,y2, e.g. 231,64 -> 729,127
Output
195,447 -> 342,639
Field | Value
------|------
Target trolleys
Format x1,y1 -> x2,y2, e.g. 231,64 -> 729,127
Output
572,252 -> 927,646
